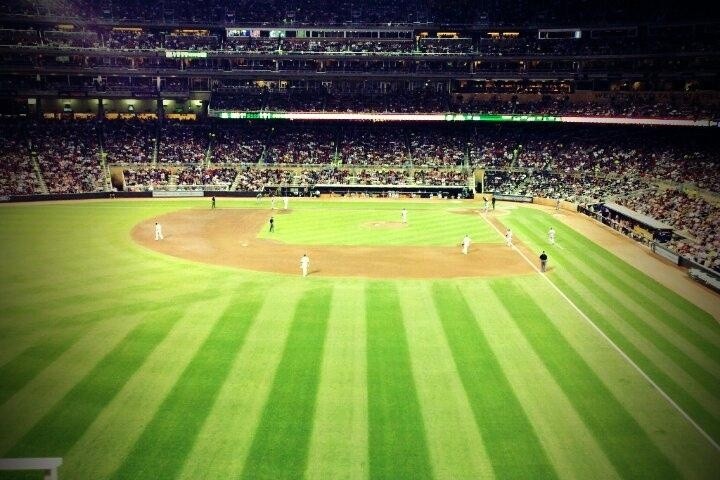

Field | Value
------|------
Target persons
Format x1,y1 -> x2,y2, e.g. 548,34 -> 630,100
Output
300,254 -> 310,276
539,250 -> 547,271
1,1 -> 720,211
270,216 -> 274,232
505,228 -> 512,246
578,186 -> 720,296
401,208 -> 407,224
548,227 -> 556,245
462,234 -> 471,255
154,222 -> 163,241
